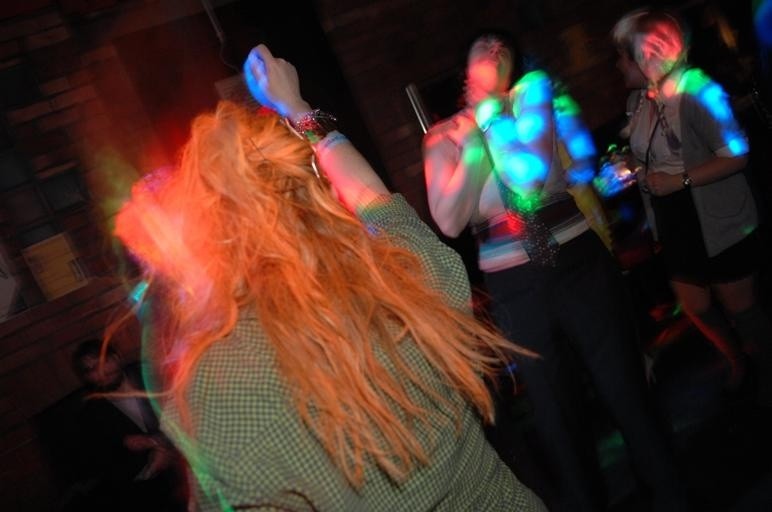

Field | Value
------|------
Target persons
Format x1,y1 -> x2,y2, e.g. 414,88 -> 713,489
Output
79,44 -> 549,512
68,339 -> 198,512
423,0 -> 772,512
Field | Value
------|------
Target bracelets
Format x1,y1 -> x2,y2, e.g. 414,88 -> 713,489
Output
292,107 -> 348,157
